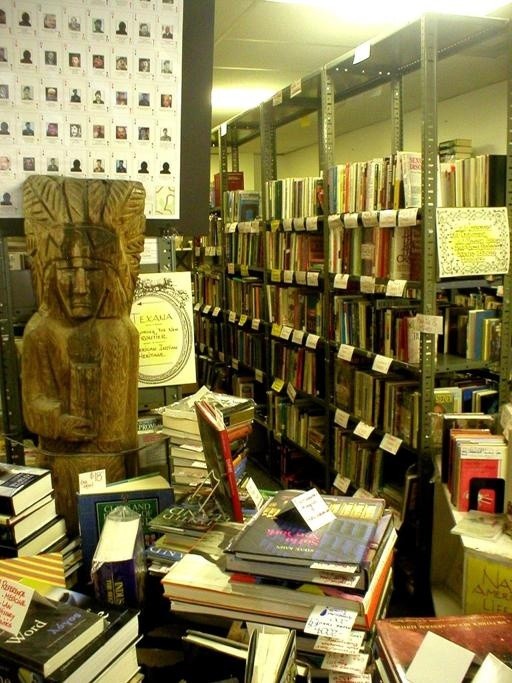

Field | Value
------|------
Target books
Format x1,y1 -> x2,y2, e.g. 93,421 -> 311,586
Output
0,385 -> 512,683
231,559 -> 233,560
178,139 -> 511,432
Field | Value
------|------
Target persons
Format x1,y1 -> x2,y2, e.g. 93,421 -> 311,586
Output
93,125 -> 104,138
22,123 -> 33,135
71,124 -> 81,136
46,124 -> 58,136
0,123 -> 12,136
115,126 -> 128,138
0,9 -> 173,110
21,180 -> 146,540
140,129 -> 149,137
158,128 -> 171,141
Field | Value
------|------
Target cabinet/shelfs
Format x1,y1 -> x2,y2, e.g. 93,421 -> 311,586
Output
325,14 -> 512,596
171,107 -> 229,392
261,67 -> 329,492
221,104 -> 265,491
431,479 -> 512,617
